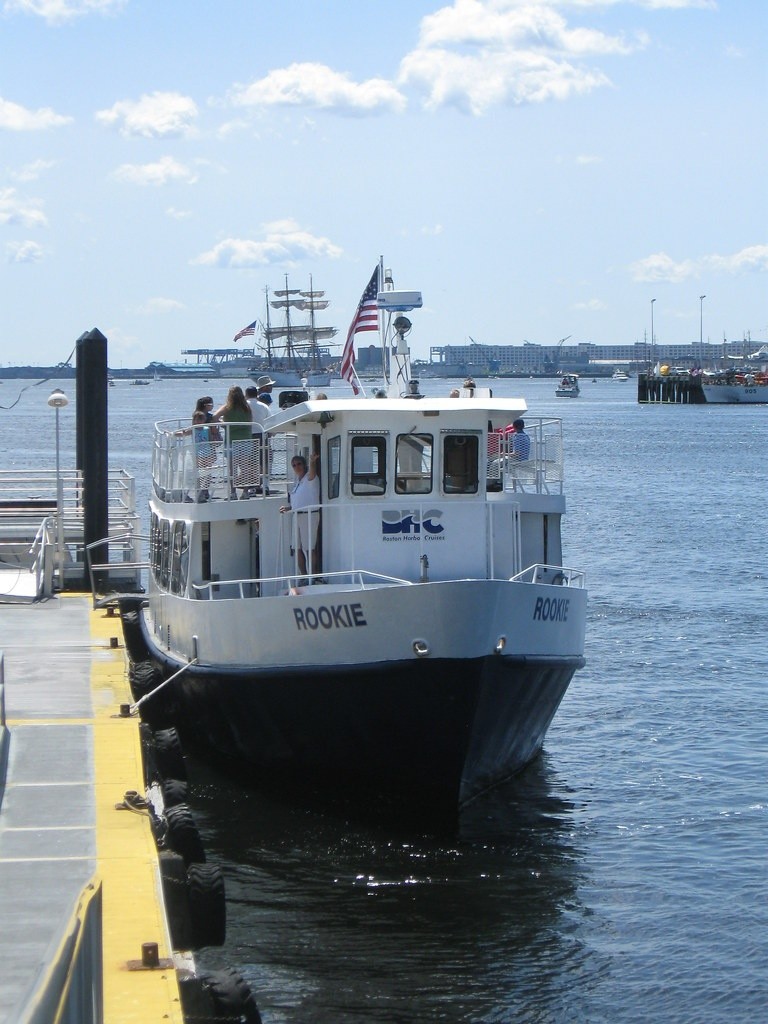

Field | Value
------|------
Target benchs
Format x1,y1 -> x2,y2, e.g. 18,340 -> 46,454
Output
510,458 -> 556,494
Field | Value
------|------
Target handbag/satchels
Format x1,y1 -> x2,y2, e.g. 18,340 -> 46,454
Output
211,431 -> 223,447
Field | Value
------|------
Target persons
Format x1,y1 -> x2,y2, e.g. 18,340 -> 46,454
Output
279,451 -> 320,586
449,379 -> 493,432
374,390 -> 388,398
192,376 -> 276,502
409,380 -> 421,394
488,419 -> 530,488
702,378 -> 746,386
316,394 -> 327,399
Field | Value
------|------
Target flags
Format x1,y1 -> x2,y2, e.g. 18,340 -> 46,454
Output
233,320 -> 256,342
340,255 -> 385,396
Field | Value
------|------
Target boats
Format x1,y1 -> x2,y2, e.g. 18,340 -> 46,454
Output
136,252 -> 587,814
128,380 -> 150,385
555,373 -> 581,398
700,382 -> 768,403
612,368 -> 628,382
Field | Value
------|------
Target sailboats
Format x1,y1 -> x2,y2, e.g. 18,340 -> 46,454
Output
246,273 -> 340,386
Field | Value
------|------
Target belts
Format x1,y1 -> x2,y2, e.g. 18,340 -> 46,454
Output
293,510 -> 318,513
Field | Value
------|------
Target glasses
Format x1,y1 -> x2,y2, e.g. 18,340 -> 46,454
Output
292,463 -> 303,467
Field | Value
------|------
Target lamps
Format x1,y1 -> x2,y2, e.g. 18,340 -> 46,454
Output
397,340 -> 408,354
393,317 -> 413,335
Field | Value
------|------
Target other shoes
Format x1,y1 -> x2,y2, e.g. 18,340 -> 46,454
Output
248,488 -> 256,496
240,491 -> 250,500
224,493 -> 238,500
199,492 -> 209,503
298,579 -> 316,587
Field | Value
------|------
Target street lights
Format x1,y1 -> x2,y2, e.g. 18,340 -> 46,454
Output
700,295 -> 706,368
650,298 -> 657,372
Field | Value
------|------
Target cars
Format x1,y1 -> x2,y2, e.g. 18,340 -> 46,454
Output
669,366 -> 688,376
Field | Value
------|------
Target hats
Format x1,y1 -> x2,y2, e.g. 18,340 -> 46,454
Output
256,376 -> 276,389
259,393 -> 273,403
